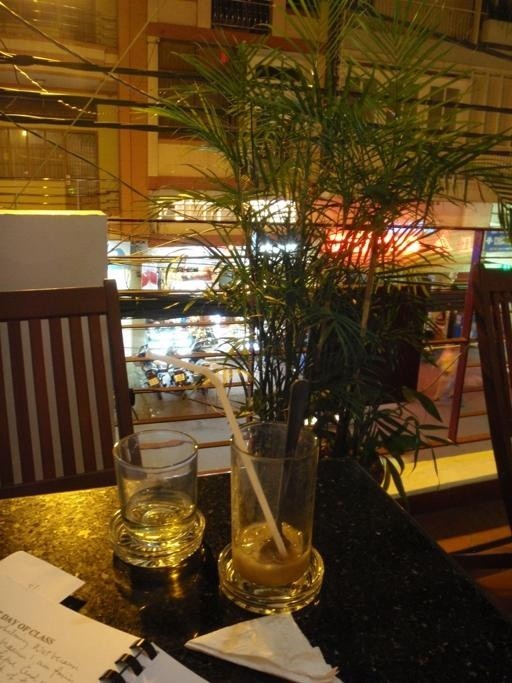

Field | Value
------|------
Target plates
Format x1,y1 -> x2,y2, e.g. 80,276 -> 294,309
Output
217,544 -> 326,617
109,511 -> 208,572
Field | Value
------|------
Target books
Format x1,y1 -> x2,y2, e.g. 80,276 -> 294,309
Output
0,574 -> 208,682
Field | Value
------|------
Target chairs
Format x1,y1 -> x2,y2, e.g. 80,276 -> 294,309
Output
468,265 -> 509,526
0,279 -> 158,496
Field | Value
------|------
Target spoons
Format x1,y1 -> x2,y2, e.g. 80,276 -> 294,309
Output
259,379 -> 311,556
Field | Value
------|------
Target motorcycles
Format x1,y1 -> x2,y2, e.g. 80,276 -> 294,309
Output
187,329 -> 215,396
161,343 -> 190,400
132,335 -> 167,400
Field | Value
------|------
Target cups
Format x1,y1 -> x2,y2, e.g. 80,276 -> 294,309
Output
226,419 -> 318,583
110,428 -> 199,544
